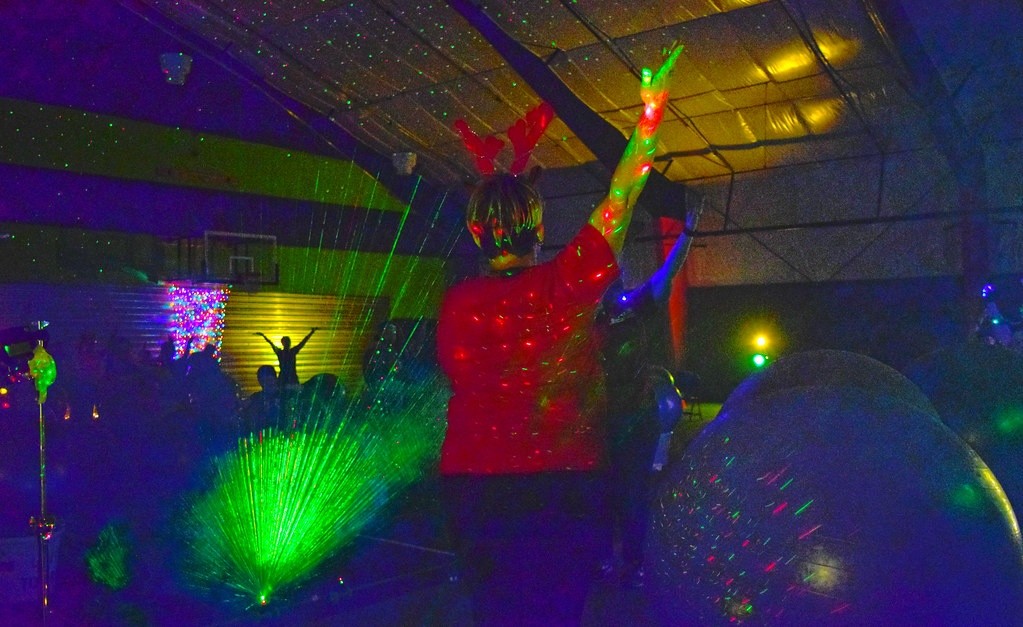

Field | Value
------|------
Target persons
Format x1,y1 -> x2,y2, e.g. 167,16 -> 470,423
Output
51,324 -> 323,436
433,34 -> 685,627
595,193 -> 715,587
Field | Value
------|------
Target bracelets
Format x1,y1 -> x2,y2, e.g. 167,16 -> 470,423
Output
682,224 -> 697,238
